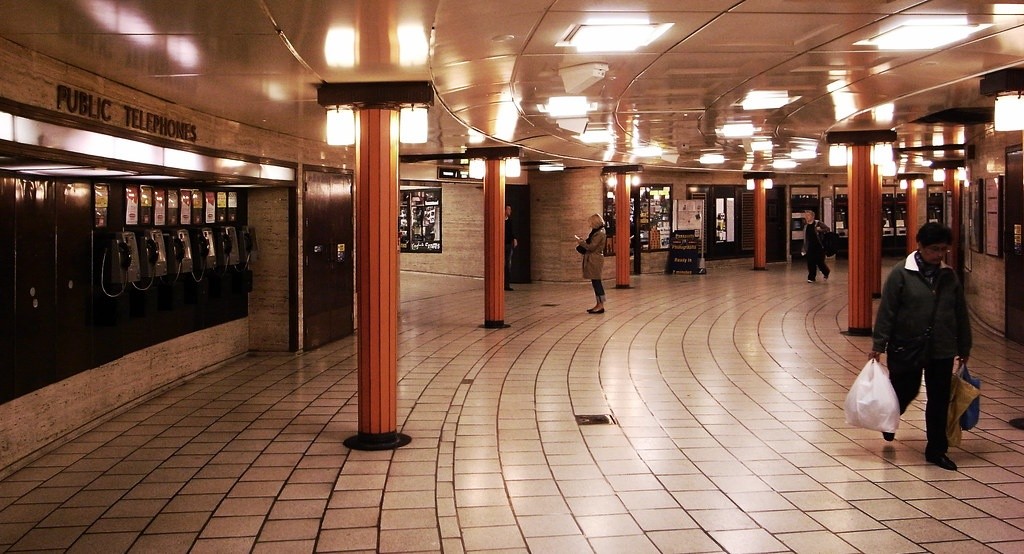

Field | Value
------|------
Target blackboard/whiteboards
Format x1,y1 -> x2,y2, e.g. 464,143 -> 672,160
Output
165,226 -> 253,273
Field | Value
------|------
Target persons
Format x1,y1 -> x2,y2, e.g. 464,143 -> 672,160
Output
867,222 -> 973,470
505,203 -> 518,291
801,208 -> 830,283
575,213 -> 607,314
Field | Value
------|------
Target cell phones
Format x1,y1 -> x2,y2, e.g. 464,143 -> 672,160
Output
575,234 -> 580,240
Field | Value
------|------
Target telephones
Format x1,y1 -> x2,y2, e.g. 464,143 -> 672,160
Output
110,230 -> 141,284
139,228 -> 169,277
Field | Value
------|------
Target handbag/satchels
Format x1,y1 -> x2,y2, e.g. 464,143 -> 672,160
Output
946,361 -> 980,448
844,358 -> 900,433
817,222 -> 841,254
887,327 -> 933,364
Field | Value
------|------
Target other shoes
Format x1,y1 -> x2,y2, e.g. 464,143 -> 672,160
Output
807,279 -> 815,283
925,450 -> 957,471
504,287 -> 514,291
1009,418 -> 1024,430
882,432 -> 896,441
587,308 -> 604,313
824,271 -> 830,280
